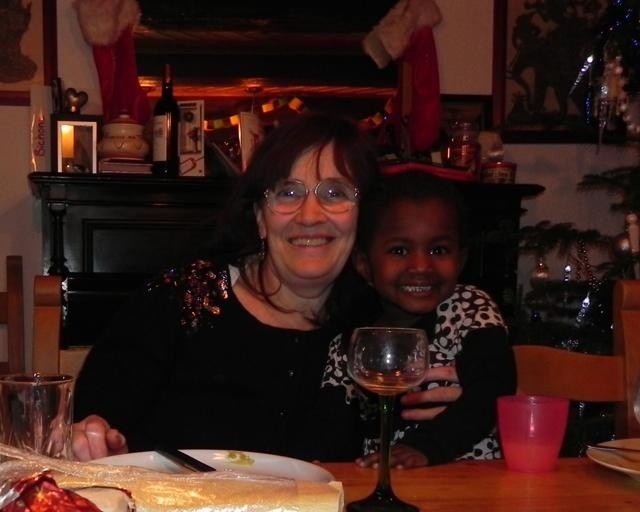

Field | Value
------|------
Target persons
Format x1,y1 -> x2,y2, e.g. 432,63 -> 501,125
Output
312,168 -> 518,468
69,117 -> 463,464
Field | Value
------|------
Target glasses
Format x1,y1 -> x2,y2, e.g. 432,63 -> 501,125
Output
263,177 -> 361,214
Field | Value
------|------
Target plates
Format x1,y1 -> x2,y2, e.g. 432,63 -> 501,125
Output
583,437 -> 640,482
88,448 -> 338,484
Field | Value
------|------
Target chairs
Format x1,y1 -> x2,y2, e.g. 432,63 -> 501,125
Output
31,270 -> 94,398
515,275 -> 640,447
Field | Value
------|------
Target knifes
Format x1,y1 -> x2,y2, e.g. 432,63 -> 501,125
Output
151,442 -> 215,475
581,442 -> 640,455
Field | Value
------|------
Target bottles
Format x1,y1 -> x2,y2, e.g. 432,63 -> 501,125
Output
533,254 -> 552,300
152,62 -> 180,177
446,121 -> 482,185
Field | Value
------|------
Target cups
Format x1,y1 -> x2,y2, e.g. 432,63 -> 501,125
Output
1,372 -> 75,461
496,394 -> 570,469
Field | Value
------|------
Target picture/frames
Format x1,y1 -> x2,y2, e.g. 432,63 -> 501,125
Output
49,111 -> 103,179
491,0 -> 639,147
1,1 -> 61,107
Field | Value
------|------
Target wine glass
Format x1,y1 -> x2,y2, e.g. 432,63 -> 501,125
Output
344,326 -> 428,511
